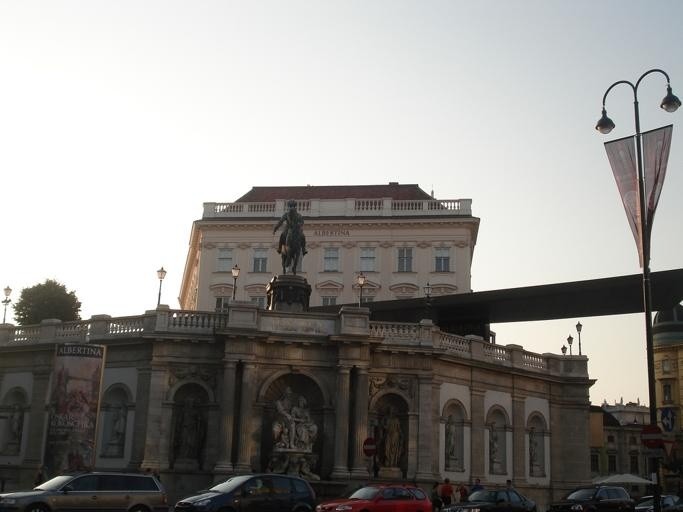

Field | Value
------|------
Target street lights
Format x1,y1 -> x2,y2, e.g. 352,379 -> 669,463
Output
356,270 -> 365,306
575,321 -> 582,355
230,263 -> 240,303
595,69 -> 682,512
155,267 -> 165,304
65,340 -> 107,468
0,285 -> 11,322
422,282 -> 432,319
567,335 -> 574,355
561,345 -> 568,357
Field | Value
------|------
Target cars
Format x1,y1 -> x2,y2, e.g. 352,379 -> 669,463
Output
314,482 -> 432,512
634,492 -> 681,511
443,485 -> 537,512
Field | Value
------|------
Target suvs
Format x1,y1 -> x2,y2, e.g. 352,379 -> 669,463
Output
0,471 -> 167,511
549,485 -> 635,512
175,472 -> 314,511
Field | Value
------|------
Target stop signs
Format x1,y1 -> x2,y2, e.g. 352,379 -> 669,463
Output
363,438 -> 375,456
639,426 -> 664,448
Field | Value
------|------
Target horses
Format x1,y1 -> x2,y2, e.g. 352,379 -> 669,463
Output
281,206 -> 301,275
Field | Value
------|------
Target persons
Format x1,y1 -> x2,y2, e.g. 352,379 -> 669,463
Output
175,394 -> 212,456
10,403 -> 22,454
272,200 -> 308,257
505,479 -> 514,489
380,404 -> 402,468
271,387 -> 318,451
445,414 -> 456,456
430,477 -> 483,512
